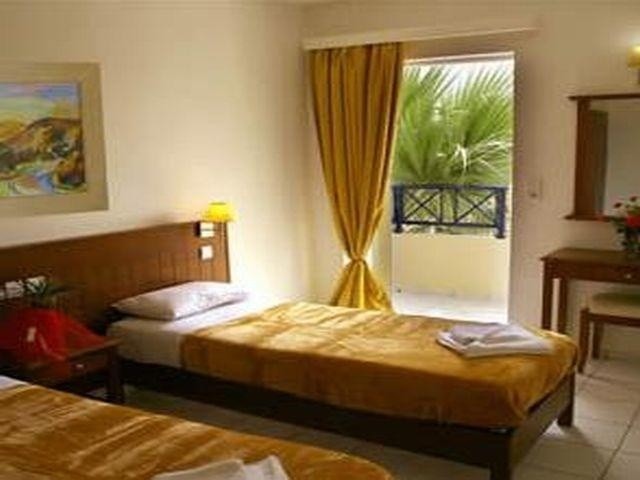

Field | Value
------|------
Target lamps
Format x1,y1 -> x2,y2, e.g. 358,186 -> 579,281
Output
199,196 -> 237,238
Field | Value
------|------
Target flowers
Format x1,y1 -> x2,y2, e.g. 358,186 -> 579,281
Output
596,190 -> 640,260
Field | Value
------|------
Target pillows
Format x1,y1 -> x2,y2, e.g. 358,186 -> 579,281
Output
107,278 -> 252,322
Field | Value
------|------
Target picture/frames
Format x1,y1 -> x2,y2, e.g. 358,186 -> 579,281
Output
0,57 -> 111,221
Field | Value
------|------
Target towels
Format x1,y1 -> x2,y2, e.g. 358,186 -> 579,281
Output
147,450 -> 289,479
432,319 -> 558,361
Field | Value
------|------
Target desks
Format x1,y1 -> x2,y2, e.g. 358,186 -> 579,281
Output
537,245 -> 640,334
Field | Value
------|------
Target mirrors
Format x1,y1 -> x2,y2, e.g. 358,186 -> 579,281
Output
559,93 -> 640,222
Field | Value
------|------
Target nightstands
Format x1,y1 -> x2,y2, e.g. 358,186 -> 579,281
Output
0,337 -> 130,404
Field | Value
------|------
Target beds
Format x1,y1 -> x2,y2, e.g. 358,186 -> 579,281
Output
0,371 -> 399,480
104,285 -> 581,479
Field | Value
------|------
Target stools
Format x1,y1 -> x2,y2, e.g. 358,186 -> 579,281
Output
576,290 -> 640,375
